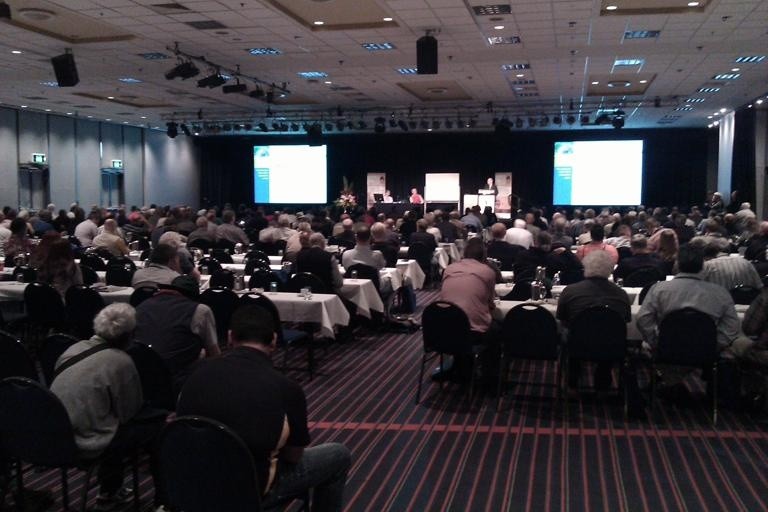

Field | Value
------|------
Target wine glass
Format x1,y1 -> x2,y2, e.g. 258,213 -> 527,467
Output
382,261 -> 386,271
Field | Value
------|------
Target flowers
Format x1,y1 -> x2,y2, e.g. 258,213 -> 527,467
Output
334,177 -> 358,214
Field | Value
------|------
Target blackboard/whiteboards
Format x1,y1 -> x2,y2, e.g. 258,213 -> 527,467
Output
425,172 -> 459,201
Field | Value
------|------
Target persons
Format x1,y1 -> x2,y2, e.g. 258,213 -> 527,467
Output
132,276 -> 223,405
52,203 -> 86,238
66,203 -> 157,262
331,205 -> 370,249
483,178 -> 498,195
150,233 -> 202,276
635,244 -> 741,391
672,190 -> 755,259
743,191 -> 768,282
596,204 -> 646,246
0,203 -> 56,265
575,224 -> 618,280
440,238 -> 517,393
738,284 -> 768,401
556,248 -> 632,392
409,188 -> 423,204
177,304 -> 350,512
615,234 -> 667,288
383,189 -> 393,203
50,303 -> 144,511
133,243 -> 180,286
567,205 -> 597,248
39,238 -> 84,289
481,191 -> 572,284
145,203 -> 333,244
700,239 -> 764,295
645,207 -> 679,262
280,223 -> 313,264
291,233 -> 341,292
369,206 -> 500,268
342,223 -> 399,322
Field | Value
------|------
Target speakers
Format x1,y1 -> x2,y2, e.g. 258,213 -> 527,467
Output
307,125 -> 323,147
166,122 -> 178,138
51,53 -> 80,86
612,116 -> 625,129
416,35 -> 438,74
493,118 -> 513,139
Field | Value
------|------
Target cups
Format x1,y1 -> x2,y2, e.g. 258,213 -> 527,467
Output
351,271 -> 357,279
302,291 -> 312,301
278,251 -> 283,257
300,289 -> 308,297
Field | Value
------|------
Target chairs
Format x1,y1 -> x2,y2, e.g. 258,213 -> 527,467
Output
105,256 -> 137,287
495,303 -> 566,411
199,287 -> 240,345
231,292 -> 315,383
638,306 -> 717,427
126,338 -> 178,406
198,257 -> 222,276
288,272 -> 327,293
149,415 -> 310,512
414,299 -> 491,406
38,333 -> 80,389
65,283 -> 107,333
0,330 -> 40,385
1,377 -> 140,512
560,305 -> 628,422
24,280 -> 64,330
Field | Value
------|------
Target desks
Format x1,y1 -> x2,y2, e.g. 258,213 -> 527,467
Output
202,251 -> 425,313
74,258 -> 402,330
494,300 -> 757,341
0,281 -> 349,386
0,266 -> 384,346
372,203 -> 423,219
494,283 -> 675,303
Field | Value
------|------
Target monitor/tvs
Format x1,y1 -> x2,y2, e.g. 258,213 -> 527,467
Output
374,194 -> 384,201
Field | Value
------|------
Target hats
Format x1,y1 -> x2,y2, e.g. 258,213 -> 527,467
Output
171,275 -> 200,293
